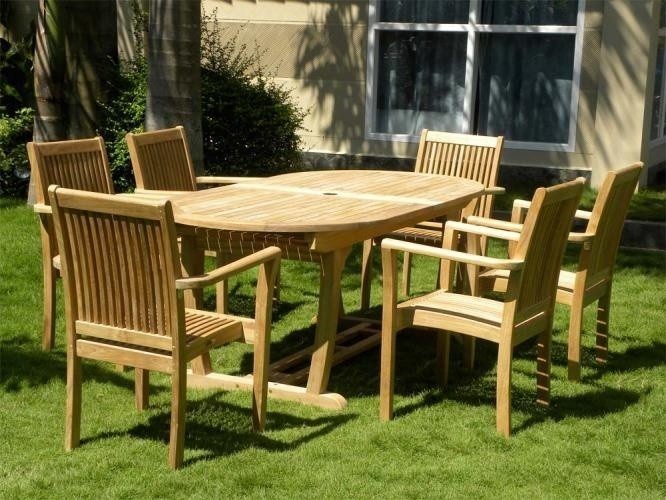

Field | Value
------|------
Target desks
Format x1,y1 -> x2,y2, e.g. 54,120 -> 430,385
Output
170,169 -> 485,413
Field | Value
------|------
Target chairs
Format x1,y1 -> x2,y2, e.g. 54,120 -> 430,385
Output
378,174 -> 587,439
467,160 -> 643,380
26,135 -> 116,354
47,184 -> 281,471
358,127 -> 505,316
125,124 -> 229,316
